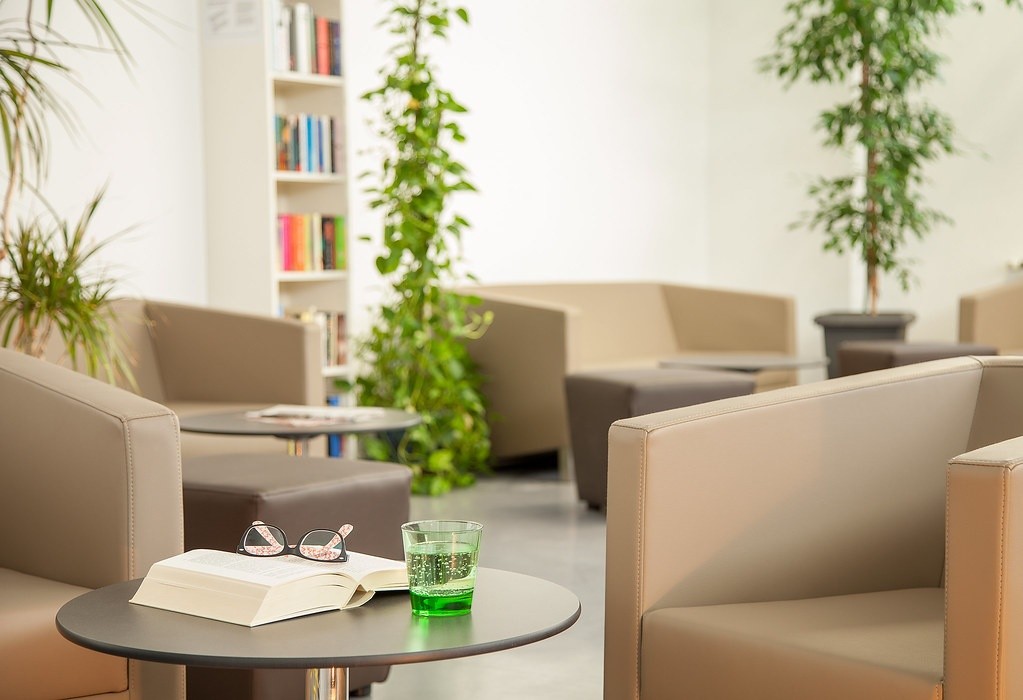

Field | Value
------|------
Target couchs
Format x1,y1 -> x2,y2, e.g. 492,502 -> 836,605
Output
450,278 -> 796,481
0,345 -> 185,700
603,354 -> 1023,700
0,296 -> 328,457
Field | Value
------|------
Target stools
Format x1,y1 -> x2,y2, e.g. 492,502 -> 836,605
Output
563,370 -> 756,517
838,338 -> 998,375
180,452 -> 414,700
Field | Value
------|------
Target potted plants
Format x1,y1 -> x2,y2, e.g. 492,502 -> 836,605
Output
333,0 -> 491,496
758,0 -> 1023,378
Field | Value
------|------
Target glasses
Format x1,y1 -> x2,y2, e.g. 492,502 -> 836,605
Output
235,519 -> 354,563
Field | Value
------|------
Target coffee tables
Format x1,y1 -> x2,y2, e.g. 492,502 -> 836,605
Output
660,351 -> 830,376
179,406 -> 421,466
55,563 -> 582,700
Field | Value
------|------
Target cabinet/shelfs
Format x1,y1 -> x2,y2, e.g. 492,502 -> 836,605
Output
200,0 -> 353,460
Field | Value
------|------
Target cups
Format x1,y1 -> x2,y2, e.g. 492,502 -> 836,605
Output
401,520 -> 484,618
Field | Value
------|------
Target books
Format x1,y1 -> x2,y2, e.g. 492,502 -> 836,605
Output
326,393 -> 354,457
278,213 -> 346,271
129,545 -> 476,626
277,3 -> 341,76
285,309 -> 348,367
275,113 -> 339,173
